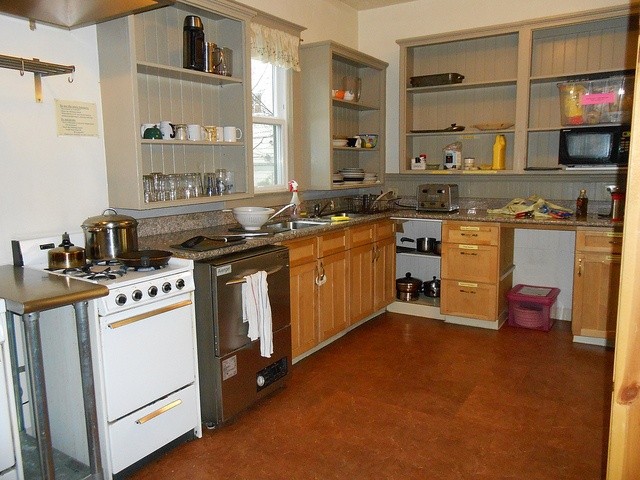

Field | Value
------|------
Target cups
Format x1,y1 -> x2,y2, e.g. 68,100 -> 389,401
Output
224,127 -> 242,142
174,125 -> 188,140
203,42 -> 221,72
612,193 -> 624,219
160,121 -> 175,139
202,126 -> 217,142
217,128 -> 223,140
218,48 -> 233,77
143,169 -> 233,202
344,73 -> 362,102
186,125 -> 205,140
140,123 -> 159,138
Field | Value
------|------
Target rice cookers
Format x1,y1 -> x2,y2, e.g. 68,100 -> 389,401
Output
442,145 -> 462,170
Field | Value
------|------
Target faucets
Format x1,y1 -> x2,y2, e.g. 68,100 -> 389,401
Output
268,203 -> 296,221
315,200 -> 333,217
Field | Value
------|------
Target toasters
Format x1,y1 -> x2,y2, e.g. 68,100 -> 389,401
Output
416,185 -> 460,211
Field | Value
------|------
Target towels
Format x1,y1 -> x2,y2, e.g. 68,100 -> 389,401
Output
242,269 -> 275,360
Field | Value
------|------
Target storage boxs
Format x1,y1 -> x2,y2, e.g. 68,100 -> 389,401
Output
507,284 -> 561,333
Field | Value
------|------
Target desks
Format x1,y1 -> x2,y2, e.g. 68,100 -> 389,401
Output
0,263 -> 108,480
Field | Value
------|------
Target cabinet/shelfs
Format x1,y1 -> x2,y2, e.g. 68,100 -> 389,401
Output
280,229 -> 348,366
571,226 -> 624,349
521,3 -> 640,173
348,218 -> 396,331
382,219 -> 442,321
397,22 -> 526,176
96,0 -> 258,211
294,39 -> 390,190
440,220 -> 514,329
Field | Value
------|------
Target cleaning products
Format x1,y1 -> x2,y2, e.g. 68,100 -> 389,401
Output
288,179 -> 301,218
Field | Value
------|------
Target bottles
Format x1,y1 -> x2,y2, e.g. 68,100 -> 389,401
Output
576,190 -> 588,219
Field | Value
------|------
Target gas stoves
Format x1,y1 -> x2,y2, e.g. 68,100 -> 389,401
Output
44,258 -> 195,289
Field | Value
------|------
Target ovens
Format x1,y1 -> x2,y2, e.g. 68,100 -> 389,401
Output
25,288 -> 202,480
195,244 -> 292,426
423,276 -> 440,297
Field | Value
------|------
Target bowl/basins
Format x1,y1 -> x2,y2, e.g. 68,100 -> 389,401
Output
347,138 -> 355,146
357,134 -> 378,147
332,140 -> 348,146
221,205 -> 275,230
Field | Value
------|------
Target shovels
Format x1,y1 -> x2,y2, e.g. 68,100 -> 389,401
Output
181,236 -> 242,248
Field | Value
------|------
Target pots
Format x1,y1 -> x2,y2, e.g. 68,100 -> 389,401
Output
115,250 -> 172,267
79,208 -> 138,262
400,237 -> 435,251
40,232 -> 86,268
396,272 -> 422,301
433,241 -> 442,254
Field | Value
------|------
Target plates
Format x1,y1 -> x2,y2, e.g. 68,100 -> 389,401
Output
334,168 -> 377,184
512,304 -> 548,327
370,190 -> 394,210
472,121 -> 515,130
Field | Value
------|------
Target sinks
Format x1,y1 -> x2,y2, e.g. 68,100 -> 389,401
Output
320,212 -> 368,221
267,219 -> 330,231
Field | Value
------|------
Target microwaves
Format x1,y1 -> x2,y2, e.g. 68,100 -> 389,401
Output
557,128 -> 628,167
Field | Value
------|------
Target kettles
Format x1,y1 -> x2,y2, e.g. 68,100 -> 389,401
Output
184,15 -> 205,70
